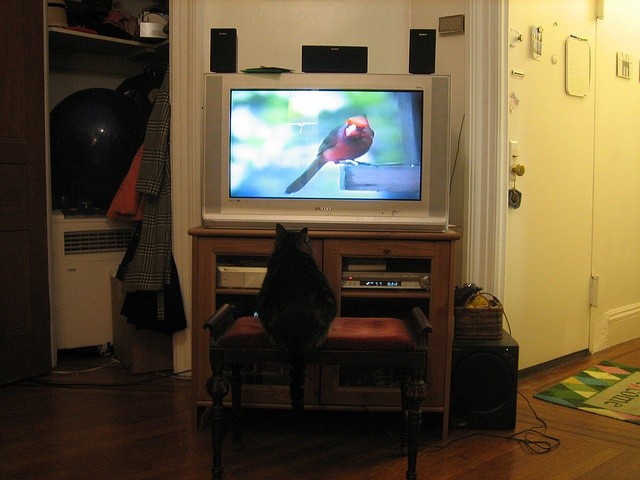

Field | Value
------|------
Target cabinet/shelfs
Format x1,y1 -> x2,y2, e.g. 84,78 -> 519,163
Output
46,24 -> 171,217
187,226 -> 462,445
1,0 -> 49,386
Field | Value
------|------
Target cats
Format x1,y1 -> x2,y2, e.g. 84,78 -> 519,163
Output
257,223 -> 335,421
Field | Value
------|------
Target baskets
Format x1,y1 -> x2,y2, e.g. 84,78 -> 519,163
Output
454,307 -> 503,341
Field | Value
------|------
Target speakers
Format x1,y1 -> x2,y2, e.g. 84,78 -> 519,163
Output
408,28 -> 437,73
448,330 -> 518,431
210,26 -> 238,72
300,44 -> 369,73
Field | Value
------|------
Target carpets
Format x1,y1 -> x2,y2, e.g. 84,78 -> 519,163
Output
532,361 -> 640,426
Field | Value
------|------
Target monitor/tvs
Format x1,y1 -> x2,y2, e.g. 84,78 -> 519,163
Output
200,72 -> 452,233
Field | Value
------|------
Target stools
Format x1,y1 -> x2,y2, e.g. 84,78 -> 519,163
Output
200,300 -> 434,480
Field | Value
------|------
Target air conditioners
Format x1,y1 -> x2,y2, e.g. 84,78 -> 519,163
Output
45,205 -> 140,366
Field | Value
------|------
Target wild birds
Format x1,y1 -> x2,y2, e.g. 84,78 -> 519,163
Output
285,113 -> 374,193
